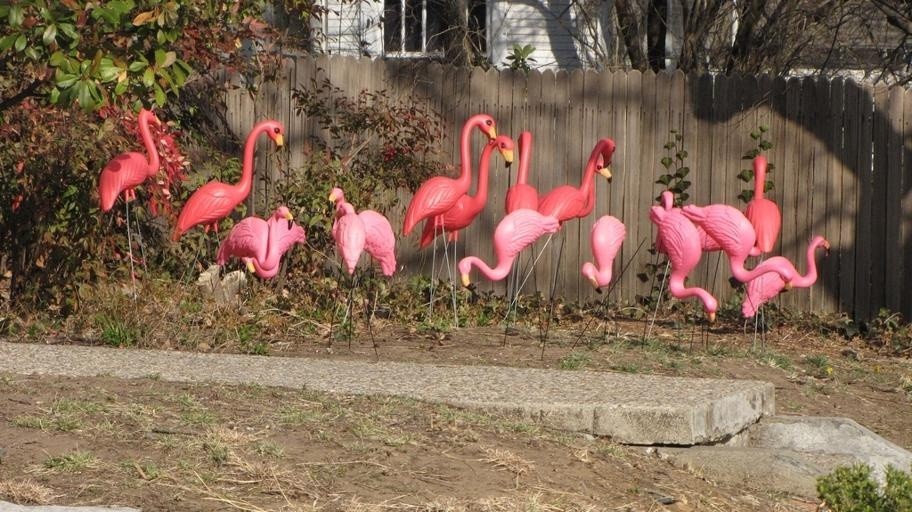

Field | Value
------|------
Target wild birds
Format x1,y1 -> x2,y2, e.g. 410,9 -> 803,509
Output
97,106 -> 161,333
579,213 -> 629,344
217,204 -> 308,314
325,184 -> 399,363
504,130 -> 616,326
399,113 -> 519,331
458,205 -> 562,346
644,154 -> 832,356
172,119 -> 285,315
